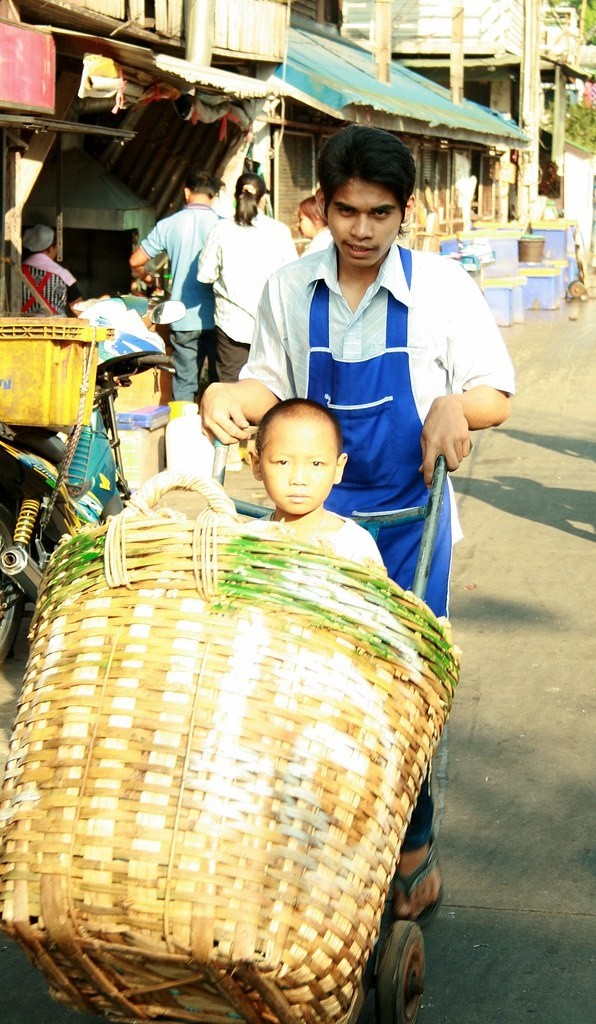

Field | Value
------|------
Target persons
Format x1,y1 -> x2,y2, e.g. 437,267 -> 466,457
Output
201,124 -> 515,919
199,174 -> 300,384
129,173 -> 227,398
246,400 -> 387,579
299,196 -> 339,259
22,224 -> 82,318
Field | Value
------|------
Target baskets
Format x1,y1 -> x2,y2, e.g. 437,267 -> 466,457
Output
0,468 -> 461,1024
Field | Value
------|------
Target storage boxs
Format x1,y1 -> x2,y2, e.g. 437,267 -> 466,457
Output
106,403 -> 171,492
110,360 -> 162,414
436,215 -> 581,330
0,315 -> 116,439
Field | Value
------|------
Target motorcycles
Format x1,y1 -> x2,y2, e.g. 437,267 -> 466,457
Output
0,301 -> 190,660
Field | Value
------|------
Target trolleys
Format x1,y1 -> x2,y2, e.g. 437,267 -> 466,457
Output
213,424 -> 447,1024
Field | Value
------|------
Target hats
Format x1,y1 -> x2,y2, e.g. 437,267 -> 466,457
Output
23,224 -> 54,251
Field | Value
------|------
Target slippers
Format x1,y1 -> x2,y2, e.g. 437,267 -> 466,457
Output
389,825 -> 445,930
